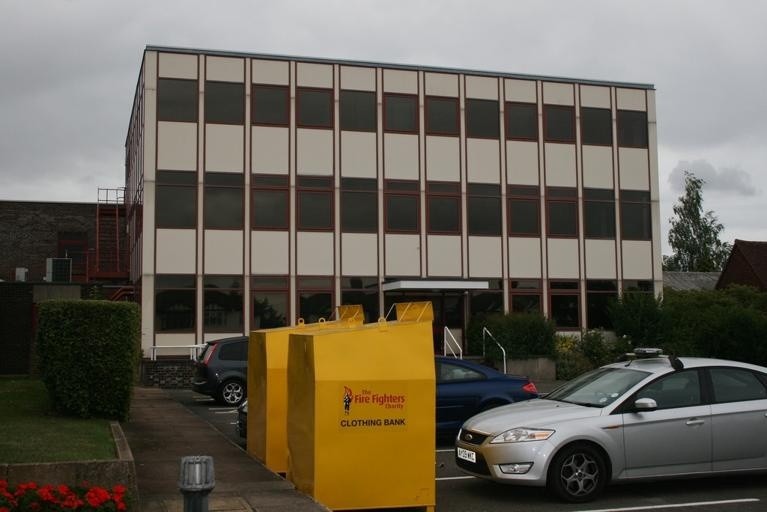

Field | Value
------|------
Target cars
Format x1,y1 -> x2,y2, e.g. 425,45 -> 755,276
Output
454,346 -> 765,504
435,353 -> 540,442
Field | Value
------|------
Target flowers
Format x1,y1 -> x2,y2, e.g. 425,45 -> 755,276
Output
0,478 -> 133,512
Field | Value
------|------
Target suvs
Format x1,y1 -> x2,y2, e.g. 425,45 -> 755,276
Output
187,336 -> 256,407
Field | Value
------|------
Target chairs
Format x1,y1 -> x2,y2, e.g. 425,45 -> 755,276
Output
639,379 -> 697,407
